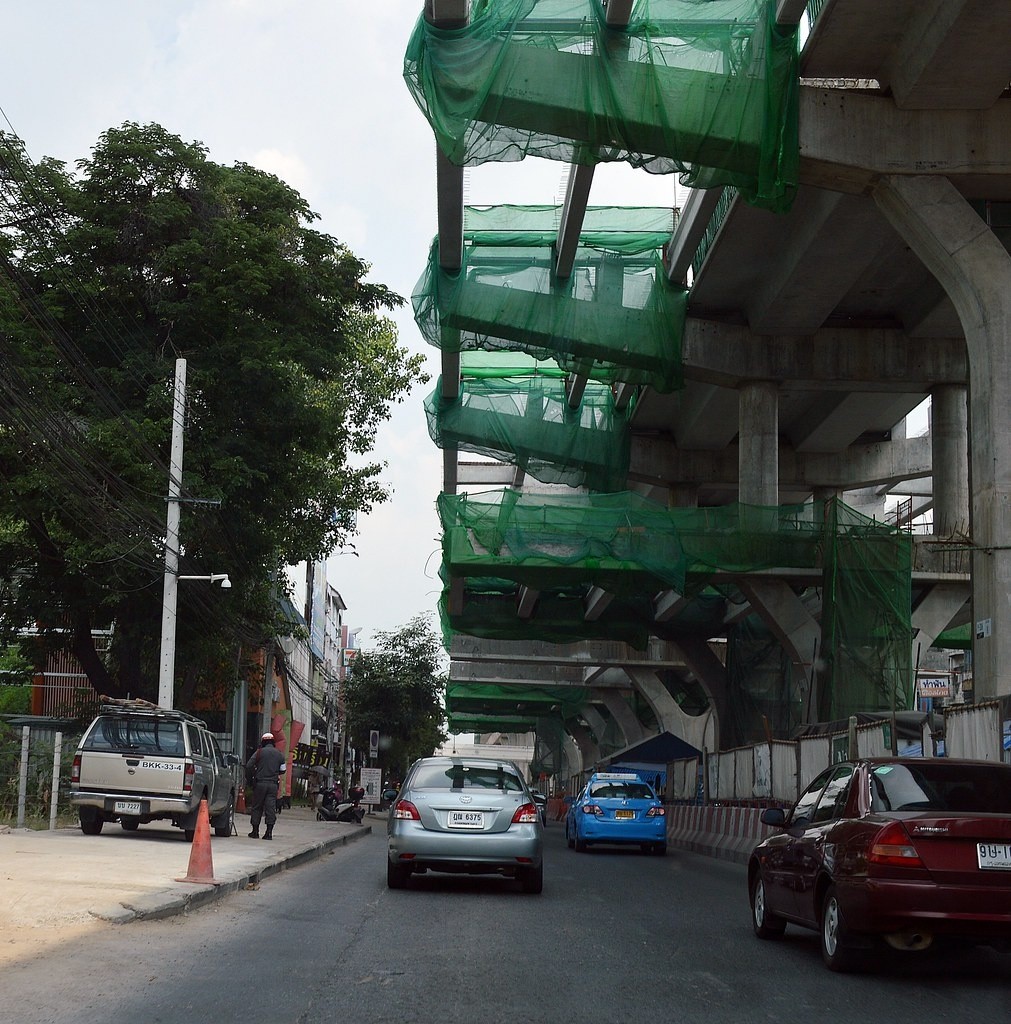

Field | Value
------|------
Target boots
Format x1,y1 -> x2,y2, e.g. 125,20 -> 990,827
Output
262,827 -> 272,840
248,825 -> 259,838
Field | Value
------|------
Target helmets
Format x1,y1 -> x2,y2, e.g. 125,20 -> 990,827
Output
262,732 -> 274,740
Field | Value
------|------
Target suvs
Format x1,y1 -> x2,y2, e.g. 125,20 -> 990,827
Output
69,711 -> 236,840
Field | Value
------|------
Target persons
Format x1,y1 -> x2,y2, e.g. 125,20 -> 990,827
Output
246,733 -> 286,839
308,771 -> 321,808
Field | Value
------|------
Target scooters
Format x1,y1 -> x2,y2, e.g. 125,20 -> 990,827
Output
313,785 -> 365,824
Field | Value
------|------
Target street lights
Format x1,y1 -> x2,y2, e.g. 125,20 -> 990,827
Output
158,573 -> 231,711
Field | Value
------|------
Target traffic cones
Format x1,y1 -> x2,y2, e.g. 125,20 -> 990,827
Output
175,800 -> 220,886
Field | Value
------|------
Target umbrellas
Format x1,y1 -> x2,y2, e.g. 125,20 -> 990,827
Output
312,766 -> 331,778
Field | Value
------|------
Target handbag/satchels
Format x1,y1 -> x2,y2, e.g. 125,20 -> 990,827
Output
251,748 -> 261,781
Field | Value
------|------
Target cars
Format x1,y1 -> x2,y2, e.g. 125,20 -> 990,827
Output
747,756 -> 1011,974
563,773 -> 669,855
380,757 -> 547,894
